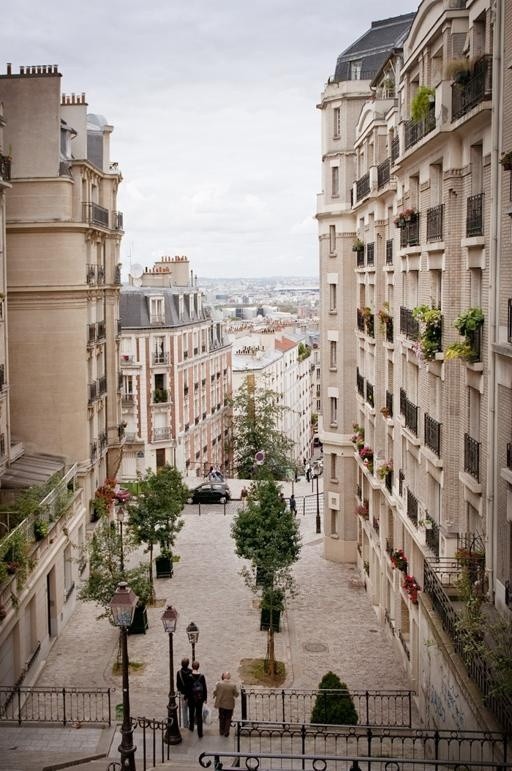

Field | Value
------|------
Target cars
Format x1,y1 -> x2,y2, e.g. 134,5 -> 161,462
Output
96,484 -> 132,506
310,453 -> 324,479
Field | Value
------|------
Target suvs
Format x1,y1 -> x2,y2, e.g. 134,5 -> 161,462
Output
184,482 -> 232,504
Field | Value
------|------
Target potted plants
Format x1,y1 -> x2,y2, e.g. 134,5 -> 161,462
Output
259,590 -> 284,633
154,549 -> 180,579
126,599 -> 148,635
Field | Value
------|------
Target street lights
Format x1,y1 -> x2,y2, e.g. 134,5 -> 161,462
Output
106,581 -> 141,771
312,463 -> 323,533
117,506 -> 125,578
185,620 -> 201,666
161,604 -> 182,744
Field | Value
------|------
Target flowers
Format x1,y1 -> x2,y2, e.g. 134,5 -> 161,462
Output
387,549 -> 422,606
414,305 -> 443,365
349,422 -> 393,481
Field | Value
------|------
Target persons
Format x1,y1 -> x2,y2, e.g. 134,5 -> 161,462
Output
306,470 -> 311,483
303,458 -> 306,466
184,660 -> 209,738
290,494 -> 297,517
212,671 -> 239,737
279,492 -> 285,502
176,656 -> 192,728
294,467 -> 298,483
